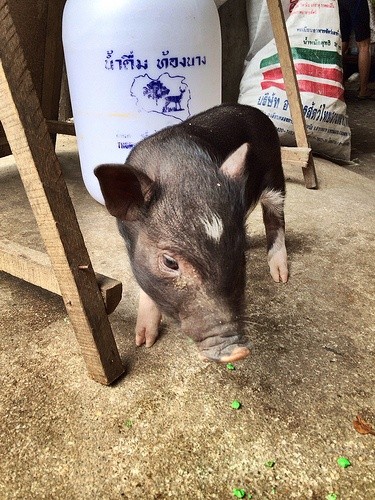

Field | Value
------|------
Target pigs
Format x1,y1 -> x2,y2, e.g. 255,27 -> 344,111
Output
94,101 -> 289,361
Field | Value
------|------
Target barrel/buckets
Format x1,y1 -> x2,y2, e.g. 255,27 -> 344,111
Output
61,0 -> 225,208
